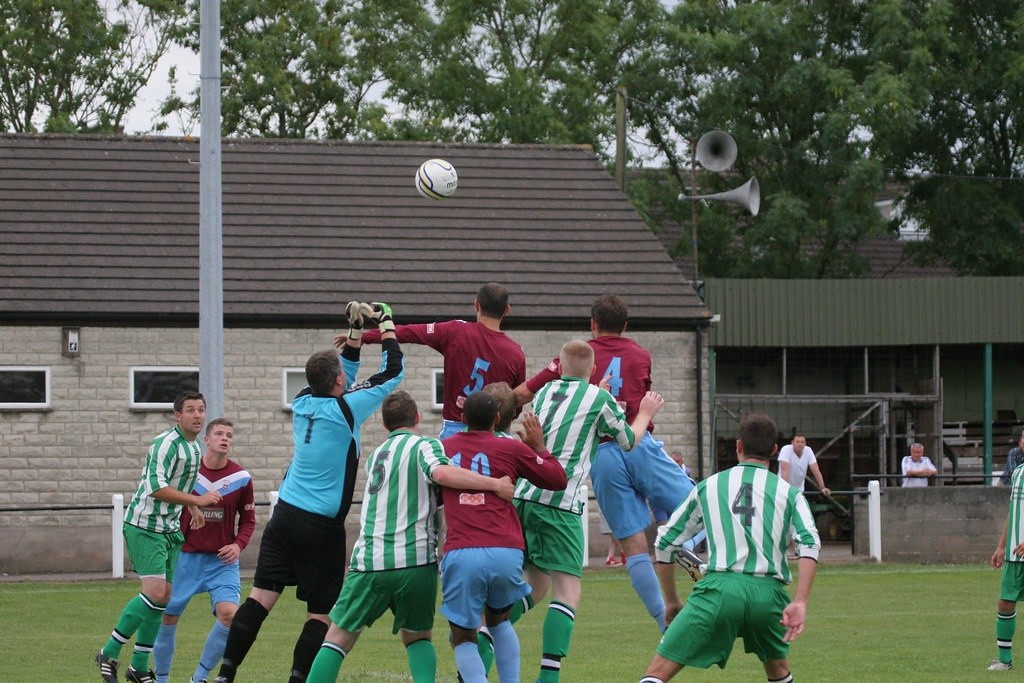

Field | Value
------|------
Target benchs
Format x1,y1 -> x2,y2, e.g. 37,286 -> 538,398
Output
905,418 -> 1024,486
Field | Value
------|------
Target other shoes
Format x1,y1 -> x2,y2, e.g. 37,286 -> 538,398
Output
189,676 -> 208,683
214,676 -> 227,683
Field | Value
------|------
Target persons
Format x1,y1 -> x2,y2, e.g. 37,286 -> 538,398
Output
214,301 -> 405,683
306,282 -> 707,682
671,450 -> 691,476
777,432 -> 832,559
595,499 -> 626,566
96,391 -> 223,682
996,431 -> 1024,489
987,462 -> 1024,671
901,443 -> 937,487
637,412 -> 820,682
153,418 -> 256,683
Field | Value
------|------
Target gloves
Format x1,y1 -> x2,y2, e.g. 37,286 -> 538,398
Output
360,301 -> 396,335
345,301 -> 364,340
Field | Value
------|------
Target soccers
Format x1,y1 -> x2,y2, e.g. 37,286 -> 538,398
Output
414,158 -> 458,201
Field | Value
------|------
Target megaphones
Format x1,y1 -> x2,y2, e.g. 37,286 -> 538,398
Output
695,129 -> 738,172
678,176 -> 761,216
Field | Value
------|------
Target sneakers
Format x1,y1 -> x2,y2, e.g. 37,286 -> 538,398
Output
95,646 -> 120,683
988,657 -> 1013,671
621,552 -> 628,566
125,663 -> 155,683
673,545 -> 707,582
605,556 -> 624,568
